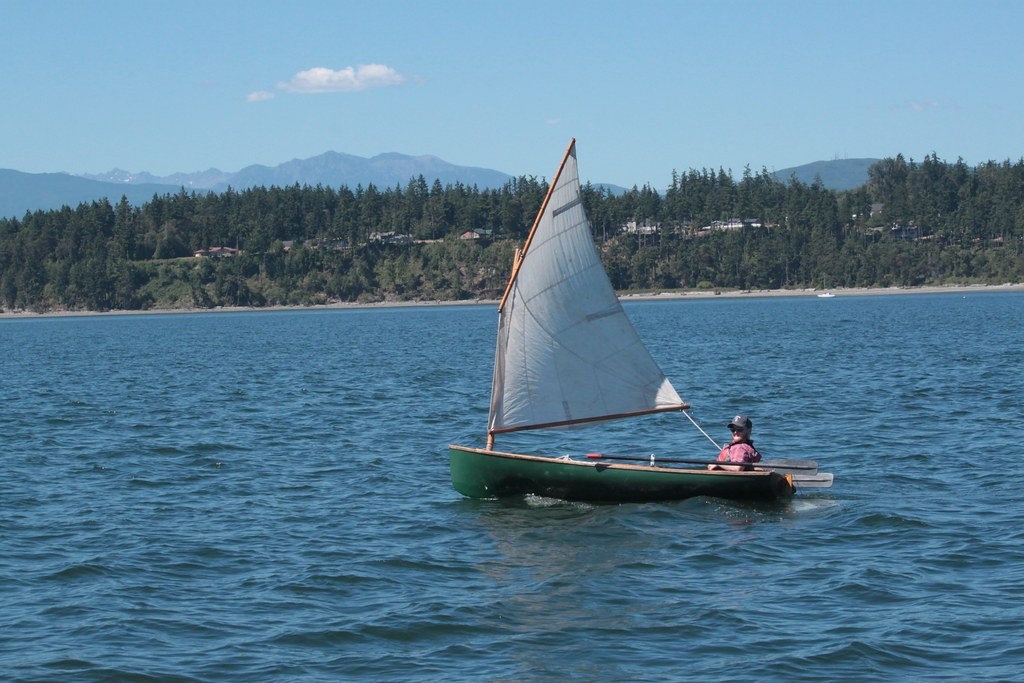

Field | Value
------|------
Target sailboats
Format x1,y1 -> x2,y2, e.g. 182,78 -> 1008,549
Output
446,137 -> 798,511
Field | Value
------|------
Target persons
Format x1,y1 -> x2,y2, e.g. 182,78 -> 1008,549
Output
707,415 -> 762,471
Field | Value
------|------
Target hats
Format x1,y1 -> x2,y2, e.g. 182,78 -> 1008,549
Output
727,416 -> 753,429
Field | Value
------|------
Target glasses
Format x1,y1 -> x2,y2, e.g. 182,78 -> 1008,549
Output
730,428 -> 746,432
